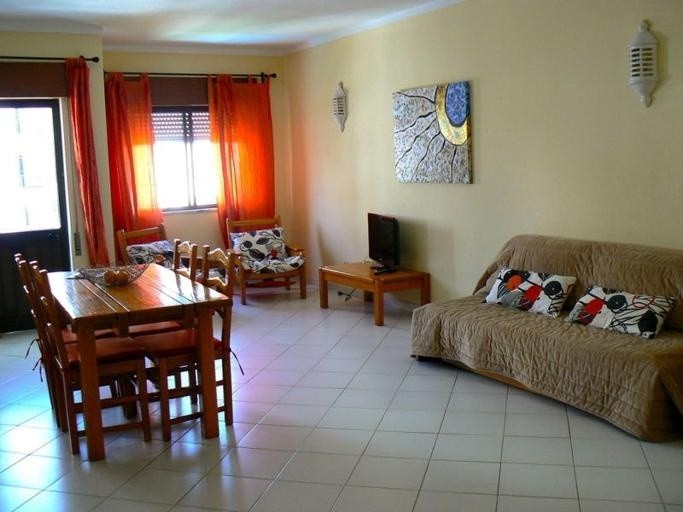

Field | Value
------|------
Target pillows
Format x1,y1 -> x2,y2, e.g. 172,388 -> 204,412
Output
476,257 -> 676,341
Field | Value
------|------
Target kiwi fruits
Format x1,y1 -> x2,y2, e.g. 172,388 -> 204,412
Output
104,271 -> 117,284
117,272 -> 128,284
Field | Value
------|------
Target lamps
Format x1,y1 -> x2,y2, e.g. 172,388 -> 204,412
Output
331,82 -> 348,132
630,17 -> 662,111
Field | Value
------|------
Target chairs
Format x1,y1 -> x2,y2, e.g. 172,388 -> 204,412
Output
225,217 -> 308,305
13,254 -> 150,447
144,238 -> 236,441
118,224 -> 231,318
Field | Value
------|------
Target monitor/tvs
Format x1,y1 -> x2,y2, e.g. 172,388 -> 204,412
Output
368,213 -> 399,274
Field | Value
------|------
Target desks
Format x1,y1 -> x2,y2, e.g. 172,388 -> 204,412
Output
45,264 -> 232,460
318,260 -> 432,328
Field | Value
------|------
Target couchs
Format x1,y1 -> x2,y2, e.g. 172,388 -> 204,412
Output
412,213 -> 683,442
